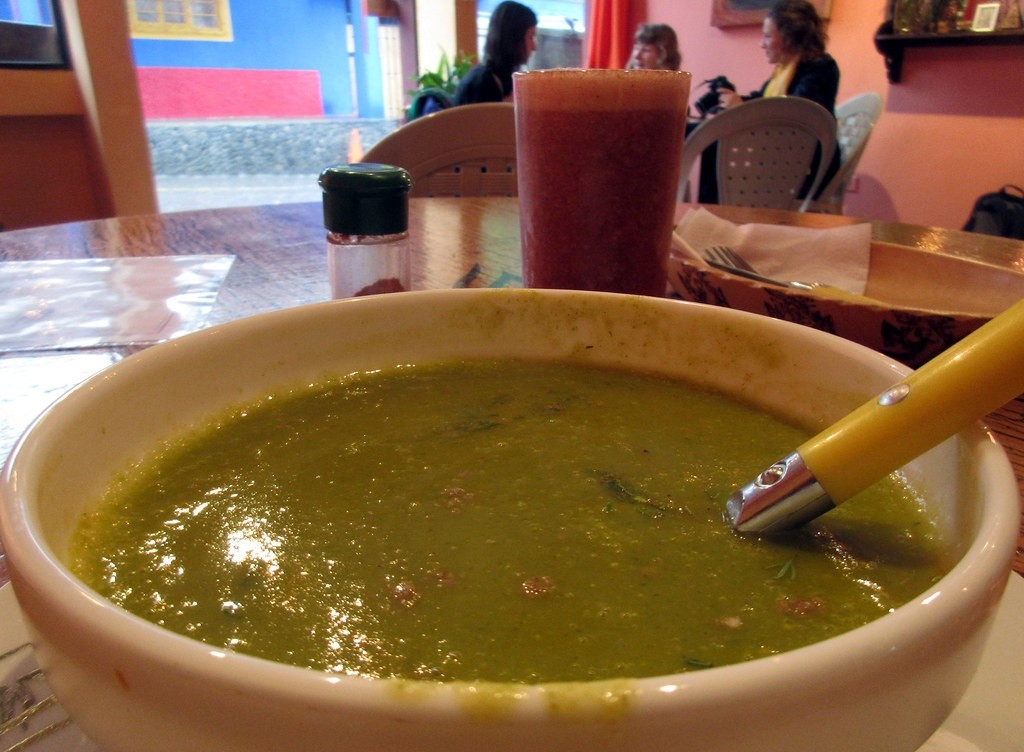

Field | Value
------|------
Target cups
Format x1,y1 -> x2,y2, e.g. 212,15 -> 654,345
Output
512,68 -> 692,301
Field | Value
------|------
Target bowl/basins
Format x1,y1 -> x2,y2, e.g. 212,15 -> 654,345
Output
0,20 -> 53,61
1,287 -> 1020,752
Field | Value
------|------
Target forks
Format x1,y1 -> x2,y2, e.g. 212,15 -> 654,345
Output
705,246 -> 757,274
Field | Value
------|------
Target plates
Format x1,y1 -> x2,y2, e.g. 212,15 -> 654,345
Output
662,236 -> 1023,371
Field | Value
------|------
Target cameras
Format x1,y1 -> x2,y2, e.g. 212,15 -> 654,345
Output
695,74 -> 736,115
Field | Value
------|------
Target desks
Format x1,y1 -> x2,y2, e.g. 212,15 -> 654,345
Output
0,201 -> 1024,752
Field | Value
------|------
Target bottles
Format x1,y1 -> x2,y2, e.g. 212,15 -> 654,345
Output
318,163 -> 411,300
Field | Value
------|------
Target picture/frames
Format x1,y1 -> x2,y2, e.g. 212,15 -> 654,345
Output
709,0 -> 833,28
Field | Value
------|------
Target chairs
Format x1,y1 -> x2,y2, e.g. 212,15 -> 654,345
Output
356,101 -> 517,198
807,93 -> 882,218
676,94 -> 839,213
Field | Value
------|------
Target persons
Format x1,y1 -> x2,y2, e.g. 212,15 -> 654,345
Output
631,24 -> 683,72
717,0 -> 844,200
455,0 -> 541,108
